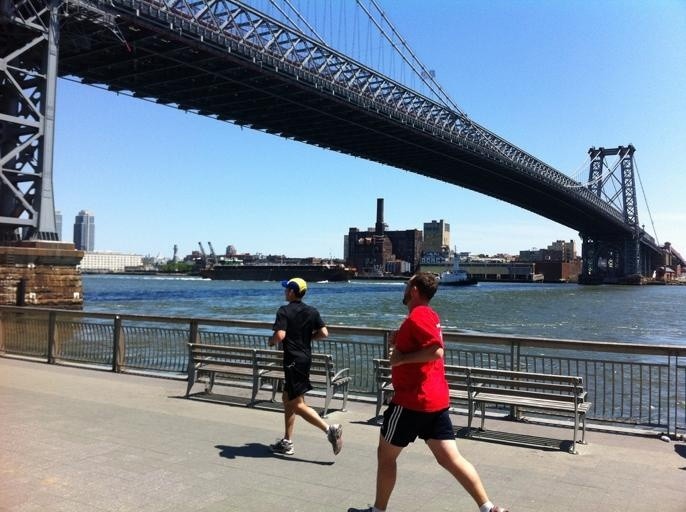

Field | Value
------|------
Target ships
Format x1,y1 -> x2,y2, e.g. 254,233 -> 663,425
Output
198,256 -> 357,283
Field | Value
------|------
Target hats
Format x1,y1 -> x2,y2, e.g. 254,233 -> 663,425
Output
281,277 -> 308,299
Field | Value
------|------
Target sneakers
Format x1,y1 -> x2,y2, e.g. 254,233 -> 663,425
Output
327,423 -> 343,455
490,504 -> 509,512
347,507 -> 374,512
269,438 -> 296,455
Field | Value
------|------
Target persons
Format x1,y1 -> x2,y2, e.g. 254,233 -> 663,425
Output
267,277 -> 342,455
349,271 -> 508,512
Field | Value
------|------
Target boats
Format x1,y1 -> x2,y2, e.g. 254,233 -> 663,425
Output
190,258 -> 243,275
125,263 -> 159,275
433,267 -> 479,286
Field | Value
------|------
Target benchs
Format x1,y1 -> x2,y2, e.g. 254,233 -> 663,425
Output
371,355 -> 594,456
184,341 -> 353,419
